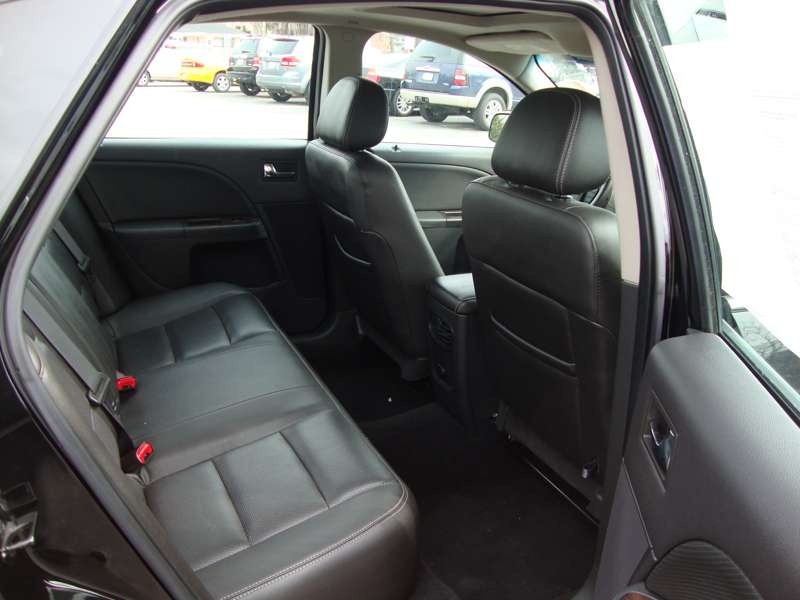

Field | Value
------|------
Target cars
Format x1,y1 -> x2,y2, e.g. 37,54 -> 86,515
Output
399,38 -> 600,131
133,30 -> 421,117
0,0 -> 800,600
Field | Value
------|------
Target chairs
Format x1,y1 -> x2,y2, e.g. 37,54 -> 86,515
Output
305,75 -> 446,380
460,87 -> 623,489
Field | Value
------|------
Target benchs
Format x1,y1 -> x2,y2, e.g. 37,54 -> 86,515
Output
20,188 -> 418,600
